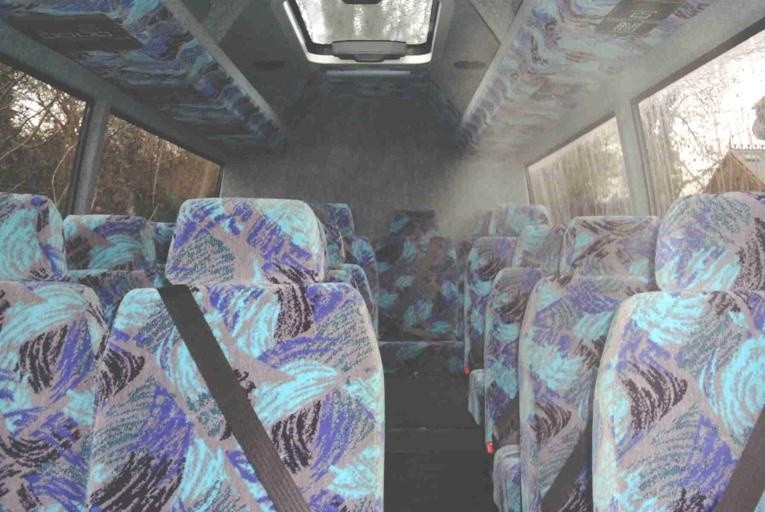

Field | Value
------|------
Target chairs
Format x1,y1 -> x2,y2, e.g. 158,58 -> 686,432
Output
374,208 -> 464,382
462,194 -> 761,510
1,192 -> 386,509
